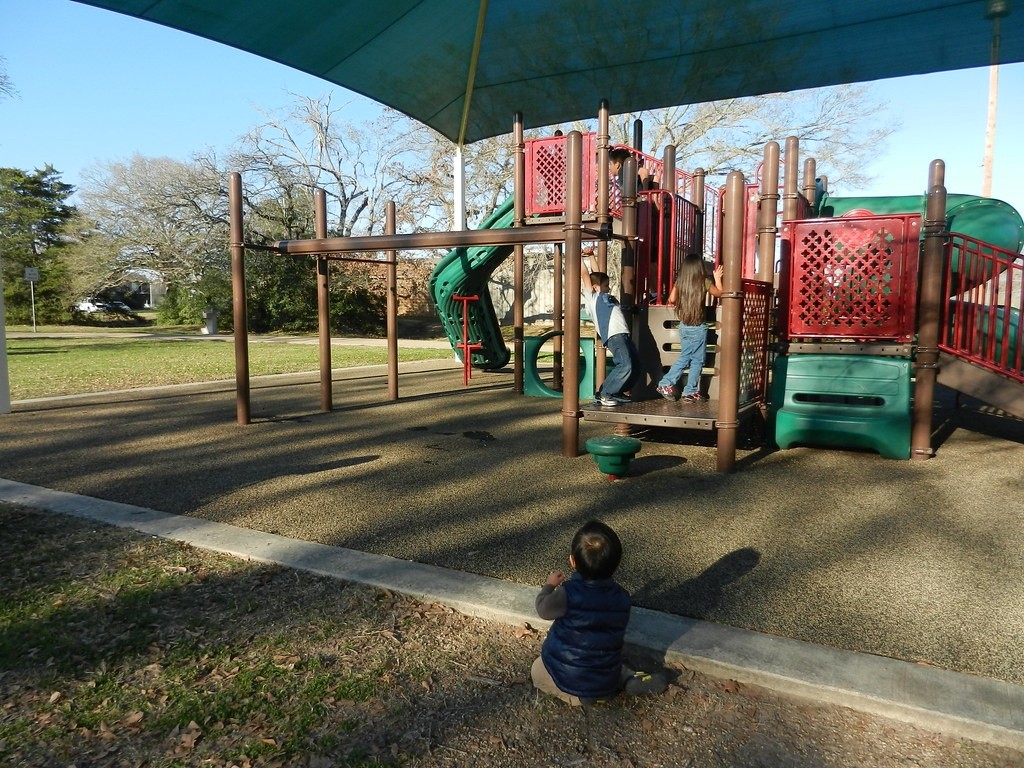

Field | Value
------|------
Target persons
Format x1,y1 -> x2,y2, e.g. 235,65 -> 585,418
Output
581,246 -> 639,406
609,149 -> 659,262
530,520 -> 671,705
656,253 -> 724,402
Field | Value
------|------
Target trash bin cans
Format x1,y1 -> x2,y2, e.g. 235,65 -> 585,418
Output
200,308 -> 217,334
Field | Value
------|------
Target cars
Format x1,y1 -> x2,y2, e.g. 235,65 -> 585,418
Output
104,301 -> 131,314
75,297 -> 107,314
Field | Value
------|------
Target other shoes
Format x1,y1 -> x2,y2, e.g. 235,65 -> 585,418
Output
575,693 -> 618,704
625,672 -> 666,696
656,386 -> 676,401
593,392 -> 617,406
682,393 -> 707,403
613,392 -> 632,402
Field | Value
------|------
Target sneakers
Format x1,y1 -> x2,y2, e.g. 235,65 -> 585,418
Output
644,293 -> 664,304
